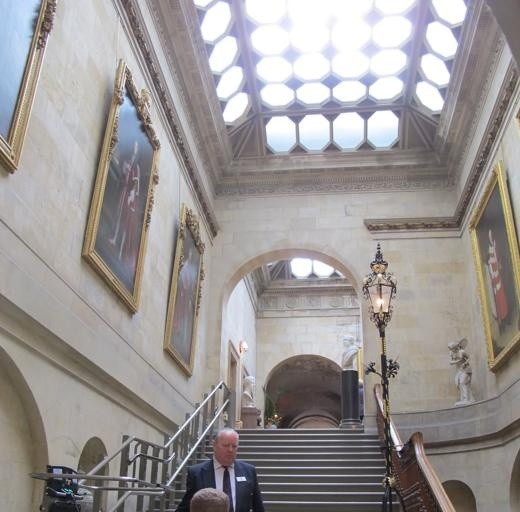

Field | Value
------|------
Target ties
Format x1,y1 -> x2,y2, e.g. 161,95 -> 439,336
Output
223,466 -> 234,512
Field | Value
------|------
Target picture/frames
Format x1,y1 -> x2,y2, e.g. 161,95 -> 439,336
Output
163,202 -> 205,377
1,0 -> 56,174
464,160 -> 520,372
81,59 -> 161,315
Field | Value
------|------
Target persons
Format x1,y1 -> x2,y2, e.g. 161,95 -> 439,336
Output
241,376 -> 256,404
485,225 -> 510,321
341,336 -> 359,370
175,427 -> 266,512
107,139 -> 142,261
190,487 -> 230,512
448,337 -> 472,405
173,247 -> 197,354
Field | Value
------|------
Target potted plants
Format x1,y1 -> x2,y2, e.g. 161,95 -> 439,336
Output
261,385 -> 289,429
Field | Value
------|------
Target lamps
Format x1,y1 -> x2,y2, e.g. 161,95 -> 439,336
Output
362,242 -> 413,511
